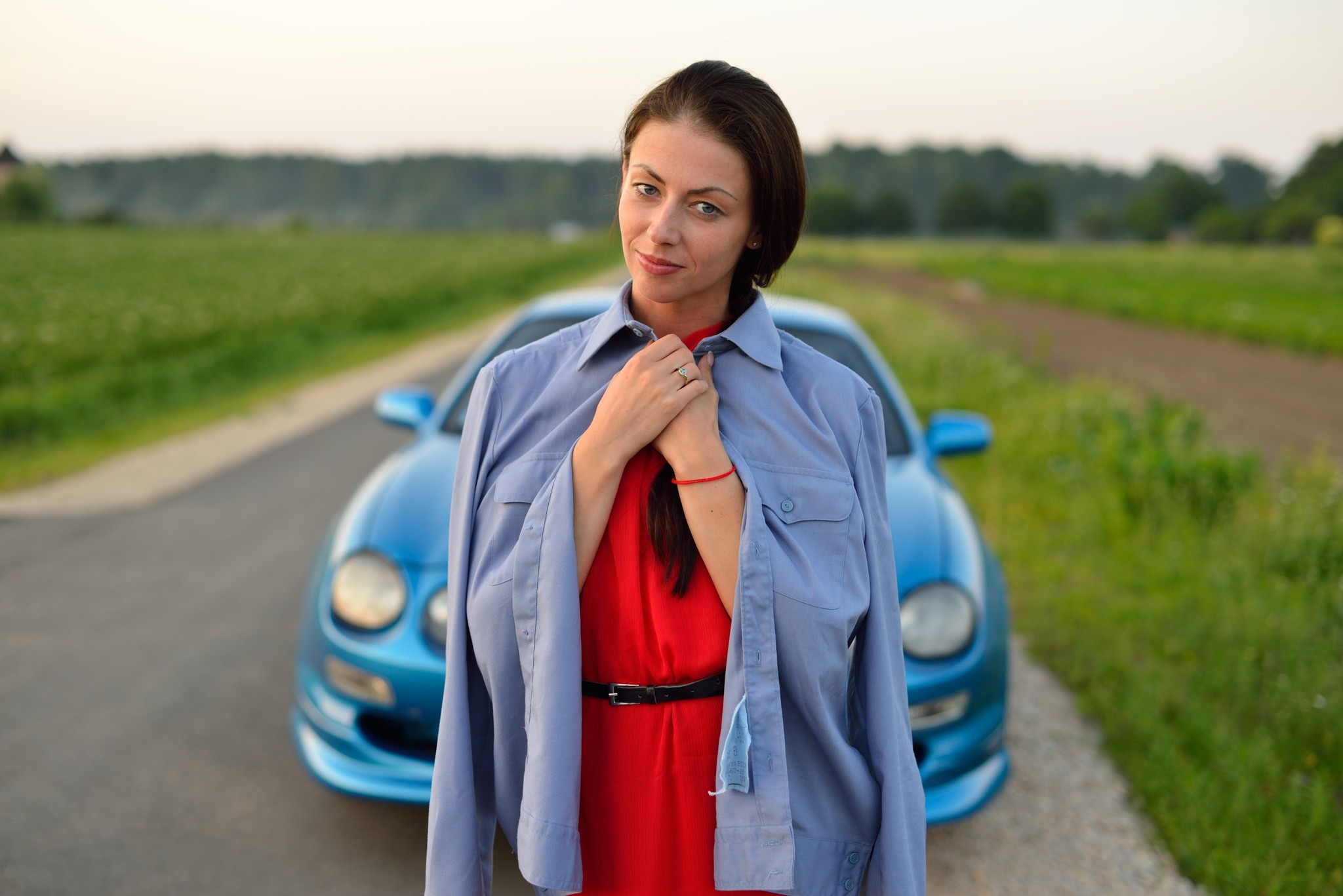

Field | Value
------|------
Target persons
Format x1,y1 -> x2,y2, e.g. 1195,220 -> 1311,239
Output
425,61 -> 925,896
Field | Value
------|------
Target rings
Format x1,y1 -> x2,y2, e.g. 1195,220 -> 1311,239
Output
675,368 -> 687,385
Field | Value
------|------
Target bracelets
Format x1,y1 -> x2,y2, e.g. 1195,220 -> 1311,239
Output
671,465 -> 736,484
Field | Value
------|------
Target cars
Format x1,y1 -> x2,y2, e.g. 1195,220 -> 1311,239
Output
291,284 -> 1014,826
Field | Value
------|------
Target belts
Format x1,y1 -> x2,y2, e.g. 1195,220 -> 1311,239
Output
581,671 -> 727,706
